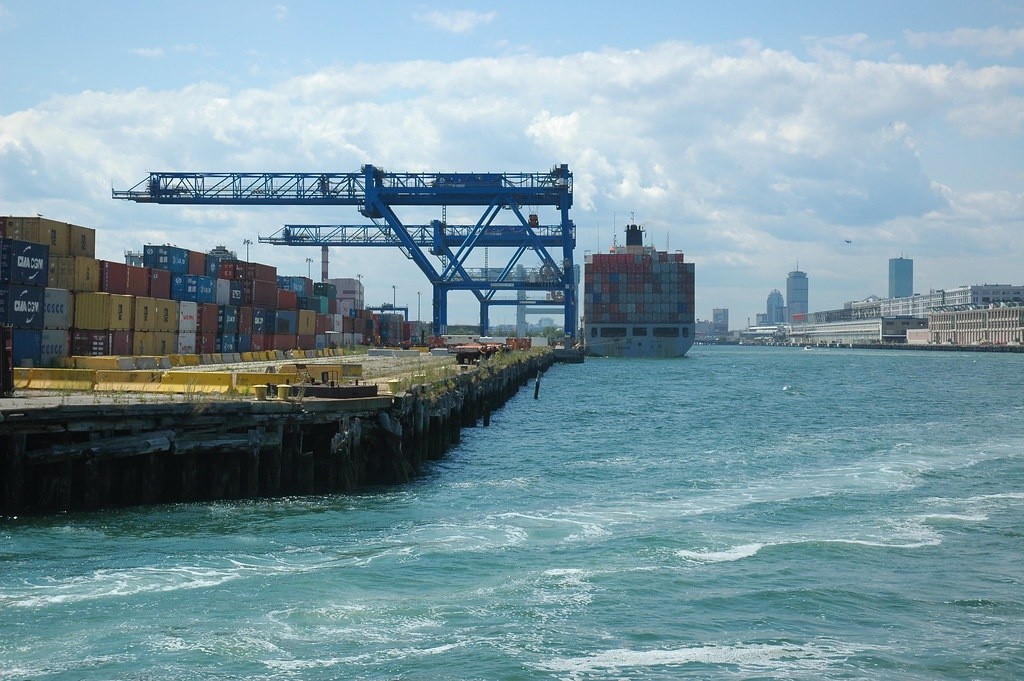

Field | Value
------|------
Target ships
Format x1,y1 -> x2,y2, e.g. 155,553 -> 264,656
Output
582,208 -> 694,361
